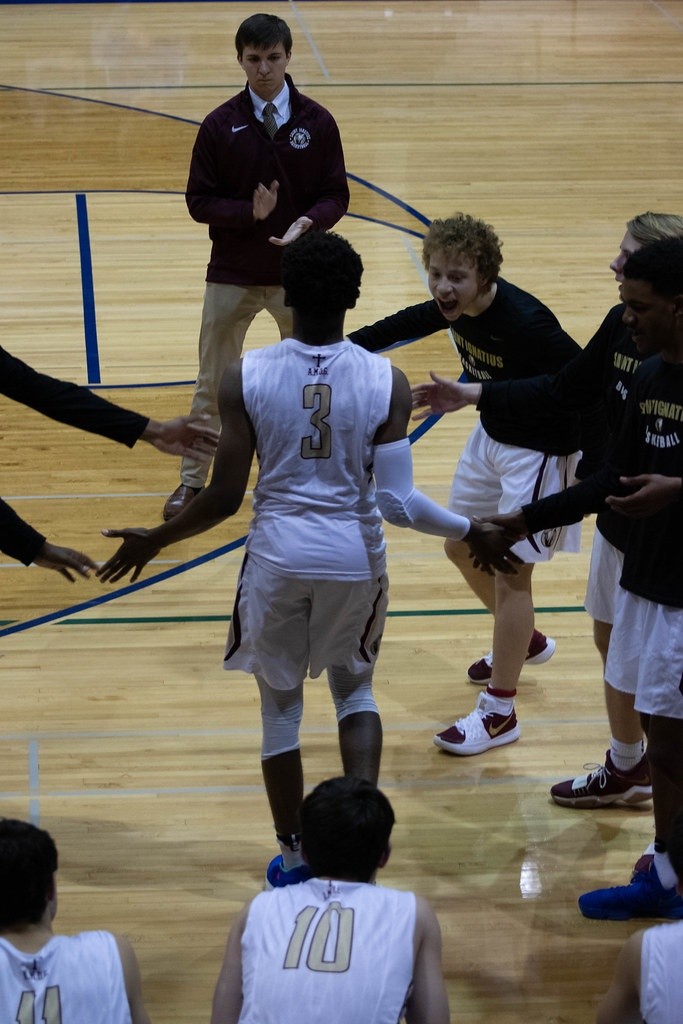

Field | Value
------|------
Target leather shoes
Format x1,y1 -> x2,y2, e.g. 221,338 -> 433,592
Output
163,483 -> 205,521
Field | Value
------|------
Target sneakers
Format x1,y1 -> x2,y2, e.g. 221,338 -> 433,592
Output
265,856 -> 315,891
468,628 -> 556,685
550,744 -> 653,808
433,690 -> 519,755
578,854 -> 683,921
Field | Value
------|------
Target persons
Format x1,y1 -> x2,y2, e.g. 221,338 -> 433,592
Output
595,815 -> 683,1024
408,211 -> 683,809
95,230 -> 525,891
0,345 -> 221,584
347,214 -> 584,755
468,237 -> 683,921
0,819 -> 152,1023
213,775 -> 452,1024
162,13 -> 350,521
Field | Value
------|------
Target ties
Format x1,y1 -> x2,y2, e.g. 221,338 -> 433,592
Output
262,103 -> 278,140
